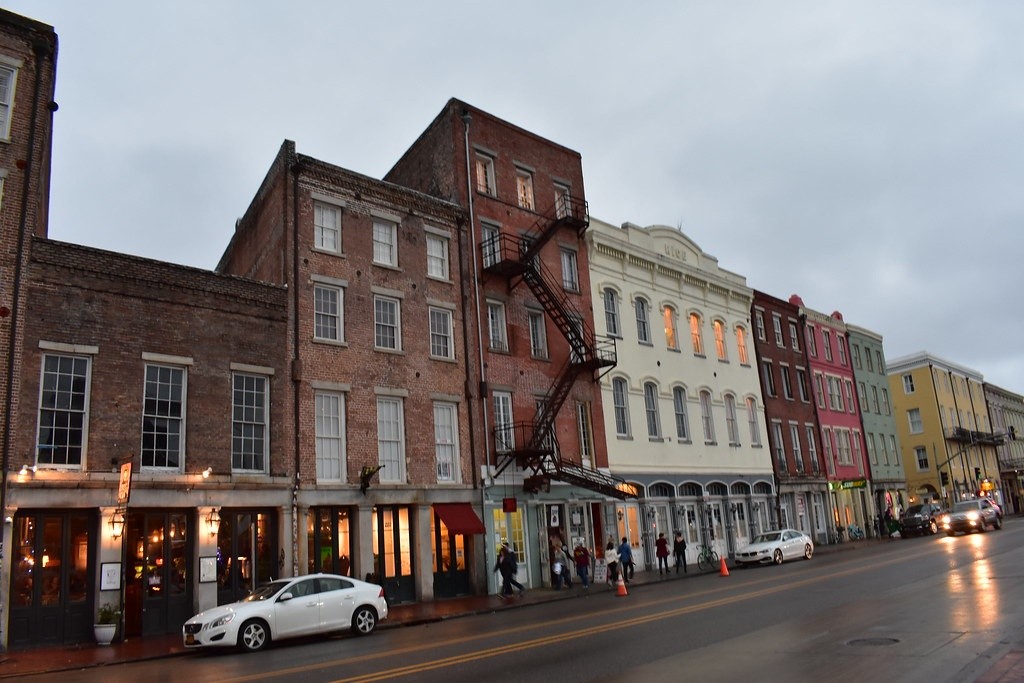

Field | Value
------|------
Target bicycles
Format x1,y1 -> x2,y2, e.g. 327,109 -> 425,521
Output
848,526 -> 865,541
695,545 -> 719,571
833,529 -> 845,544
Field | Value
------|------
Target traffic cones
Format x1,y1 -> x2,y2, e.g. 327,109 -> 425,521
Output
614,570 -> 628,595
718,556 -> 730,577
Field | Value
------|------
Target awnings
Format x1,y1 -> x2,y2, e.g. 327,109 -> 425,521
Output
432,502 -> 486,535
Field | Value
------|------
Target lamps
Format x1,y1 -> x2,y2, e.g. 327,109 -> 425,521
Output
205,507 -> 221,538
108,509 -> 126,538
19,465 -> 37,476
203,467 -> 212,480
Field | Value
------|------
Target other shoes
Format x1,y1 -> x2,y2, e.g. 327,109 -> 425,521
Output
497,593 -> 505,599
608,580 -> 613,587
666,569 -> 670,572
519,587 -> 526,595
684,569 -> 687,573
676,568 -> 679,573
660,571 -> 663,575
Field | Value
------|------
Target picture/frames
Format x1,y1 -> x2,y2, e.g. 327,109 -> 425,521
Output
100,562 -> 122,591
199,556 -> 217,583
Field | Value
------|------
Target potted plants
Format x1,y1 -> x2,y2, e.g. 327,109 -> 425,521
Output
92,604 -> 123,646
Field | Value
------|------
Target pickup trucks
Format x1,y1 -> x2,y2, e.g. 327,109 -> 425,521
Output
897,502 -> 951,539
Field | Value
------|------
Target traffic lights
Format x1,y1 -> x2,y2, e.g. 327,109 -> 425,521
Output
975,467 -> 981,480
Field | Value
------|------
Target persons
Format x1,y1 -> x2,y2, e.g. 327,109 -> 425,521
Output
617,537 -> 634,585
673,532 -> 687,574
605,542 -> 621,587
494,543 -> 525,599
549,534 -> 592,591
656,533 -> 671,576
878,508 -> 904,537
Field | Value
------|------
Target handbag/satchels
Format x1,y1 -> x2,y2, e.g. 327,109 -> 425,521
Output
665,543 -> 672,554
630,554 -> 636,565
553,563 -> 562,575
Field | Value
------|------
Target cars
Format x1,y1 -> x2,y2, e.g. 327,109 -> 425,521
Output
734,528 -> 814,566
943,498 -> 1003,536
183,570 -> 389,651
